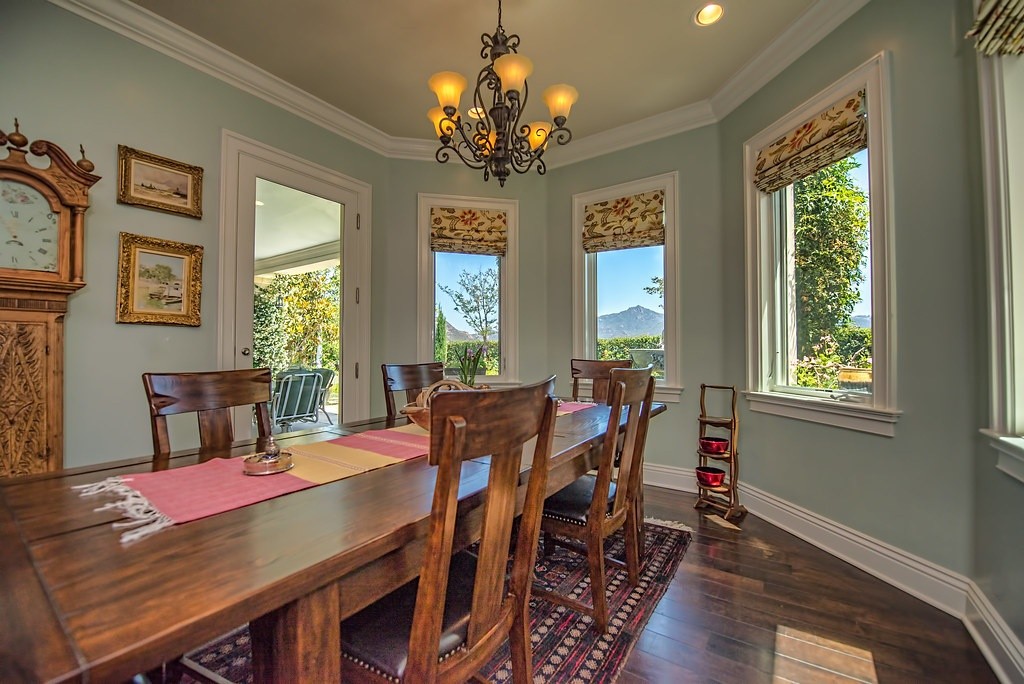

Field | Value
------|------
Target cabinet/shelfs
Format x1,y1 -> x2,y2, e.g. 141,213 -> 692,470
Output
693,383 -> 749,520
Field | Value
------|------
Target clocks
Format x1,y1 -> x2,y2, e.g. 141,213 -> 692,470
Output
0,116 -> 104,478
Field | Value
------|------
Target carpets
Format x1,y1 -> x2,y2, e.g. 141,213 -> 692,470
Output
162,516 -> 696,684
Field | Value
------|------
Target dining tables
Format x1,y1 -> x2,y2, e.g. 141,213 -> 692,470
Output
0,402 -> 668,684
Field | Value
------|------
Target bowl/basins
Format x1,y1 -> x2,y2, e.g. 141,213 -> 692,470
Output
699,437 -> 729,453
696,467 -> 725,487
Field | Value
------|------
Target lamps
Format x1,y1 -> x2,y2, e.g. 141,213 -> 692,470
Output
425,0 -> 579,189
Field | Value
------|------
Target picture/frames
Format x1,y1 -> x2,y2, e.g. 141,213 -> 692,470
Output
116,143 -> 204,220
115,231 -> 204,328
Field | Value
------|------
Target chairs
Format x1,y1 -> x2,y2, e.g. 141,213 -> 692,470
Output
337,373 -> 559,684
571,359 -> 632,405
252,368 -> 335,434
530,363 -> 656,634
381,362 -> 444,416
141,367 -> 273,455
